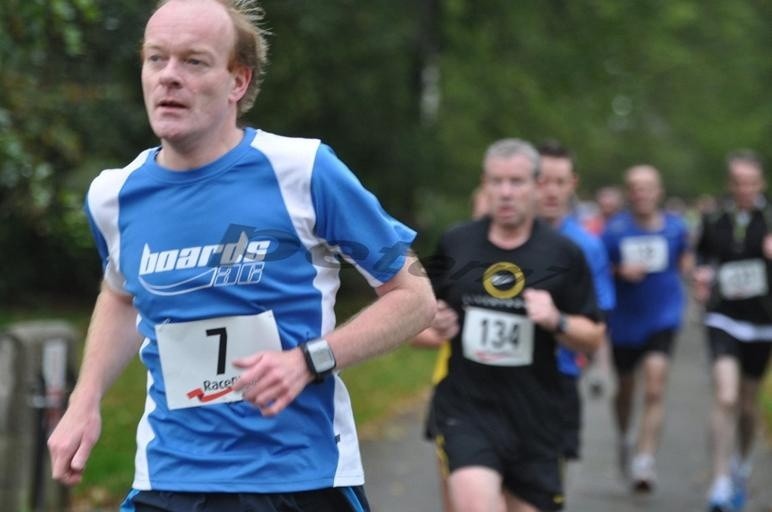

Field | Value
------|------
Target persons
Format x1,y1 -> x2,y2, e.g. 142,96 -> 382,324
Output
408,135 -> 772,511
47,0 -> 439,510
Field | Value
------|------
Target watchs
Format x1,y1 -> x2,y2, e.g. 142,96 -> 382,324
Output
300,338 -> 337,383
